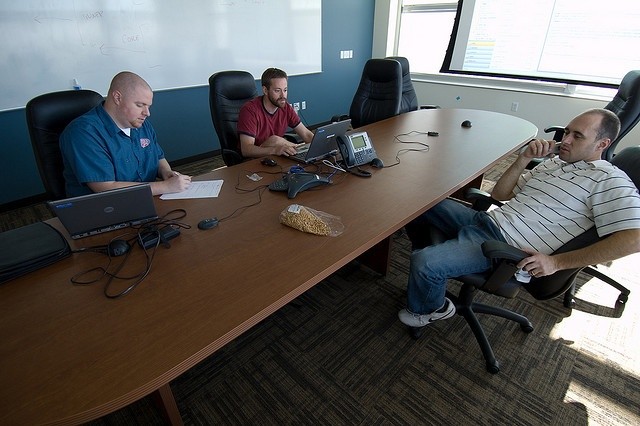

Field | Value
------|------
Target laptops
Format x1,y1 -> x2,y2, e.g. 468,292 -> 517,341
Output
282,119 -> 351,165
47,183 -> 158,241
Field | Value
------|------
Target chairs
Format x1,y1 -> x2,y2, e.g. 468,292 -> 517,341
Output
522,69 -> 640,171
384,56 -> 441,115
444,145 -> 639,373
27,89 -> 106,217
209,71 -> 304,167
331,58 -> 402,129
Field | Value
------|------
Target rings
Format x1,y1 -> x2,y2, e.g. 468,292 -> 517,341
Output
530,271 -> 535,276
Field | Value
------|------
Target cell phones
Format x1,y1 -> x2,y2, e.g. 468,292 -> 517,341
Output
549,142 -> 561,154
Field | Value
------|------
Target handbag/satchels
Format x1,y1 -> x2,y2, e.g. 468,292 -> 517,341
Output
0,222 -> 72,283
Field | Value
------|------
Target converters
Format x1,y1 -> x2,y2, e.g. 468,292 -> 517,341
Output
140,225 -> 180,249
106,239 -> 131,257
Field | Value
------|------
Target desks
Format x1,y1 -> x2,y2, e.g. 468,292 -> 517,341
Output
1,109 -> 538,425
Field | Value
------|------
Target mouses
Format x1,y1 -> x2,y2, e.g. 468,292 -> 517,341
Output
372,157 -> 383,167
198,217 -> 218,229
260,157 -> 277,167
461,120 -> 472,127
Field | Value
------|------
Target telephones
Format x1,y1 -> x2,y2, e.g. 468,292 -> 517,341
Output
336,130 -> 378,177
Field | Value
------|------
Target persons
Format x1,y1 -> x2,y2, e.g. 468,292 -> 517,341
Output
237,68 -> 315,159
397,109 -> 640,328
59,71 -> 191,197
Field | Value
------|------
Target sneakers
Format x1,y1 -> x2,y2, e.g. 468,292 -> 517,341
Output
399,296 -> 457,328
